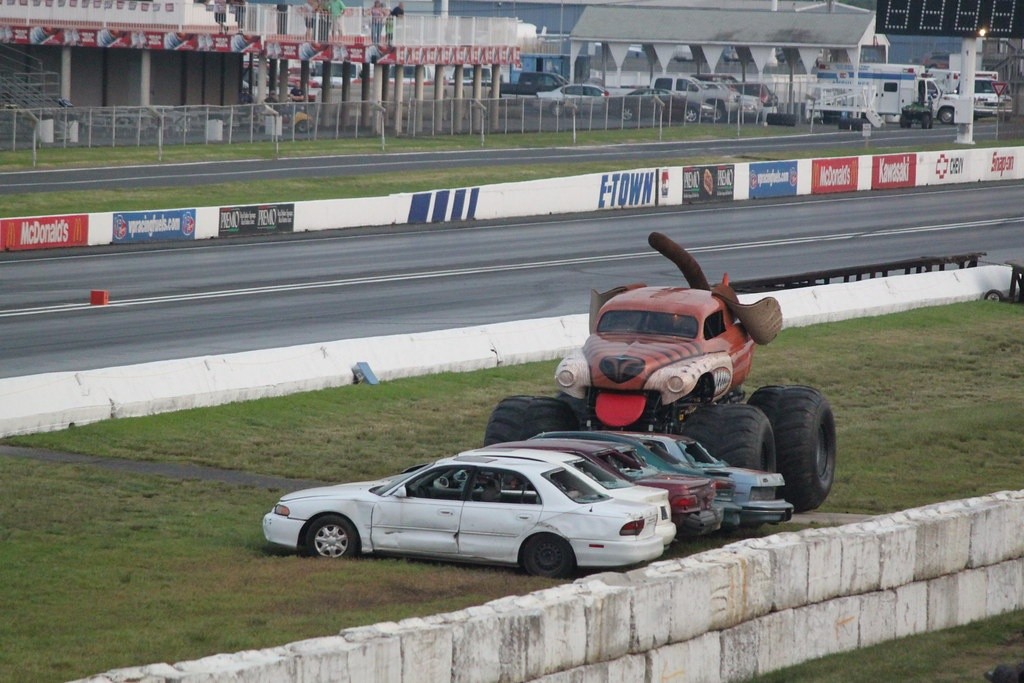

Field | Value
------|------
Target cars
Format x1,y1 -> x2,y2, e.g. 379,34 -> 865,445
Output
388,65 -> 432,84
602,87 -> 721,123
605,431 -> 795,530
692,75 -> 773,122
484,432 -> 742,537
534,83 -> 607,118
448,64 -> 491,85
310,60 -> 362,88
261,450 -> 677,579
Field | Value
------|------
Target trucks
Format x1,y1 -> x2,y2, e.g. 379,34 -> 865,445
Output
815,59 -> 959,125
927,67 -> 1013,120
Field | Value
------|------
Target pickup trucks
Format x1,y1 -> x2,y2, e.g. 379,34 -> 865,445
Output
604,75 -> 741,120
500,71 -> 569,94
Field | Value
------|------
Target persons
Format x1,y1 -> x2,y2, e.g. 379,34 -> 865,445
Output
239,85 -> 254,103
203,0 -> 245,36
289,80 -> 305,113
298,0 -> 347,43
265,90 -> 281,111
366,0 -> 405,45
276,4 -> 292,34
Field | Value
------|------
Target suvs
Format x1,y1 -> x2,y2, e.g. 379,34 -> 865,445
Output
484,282 -> 836,519
